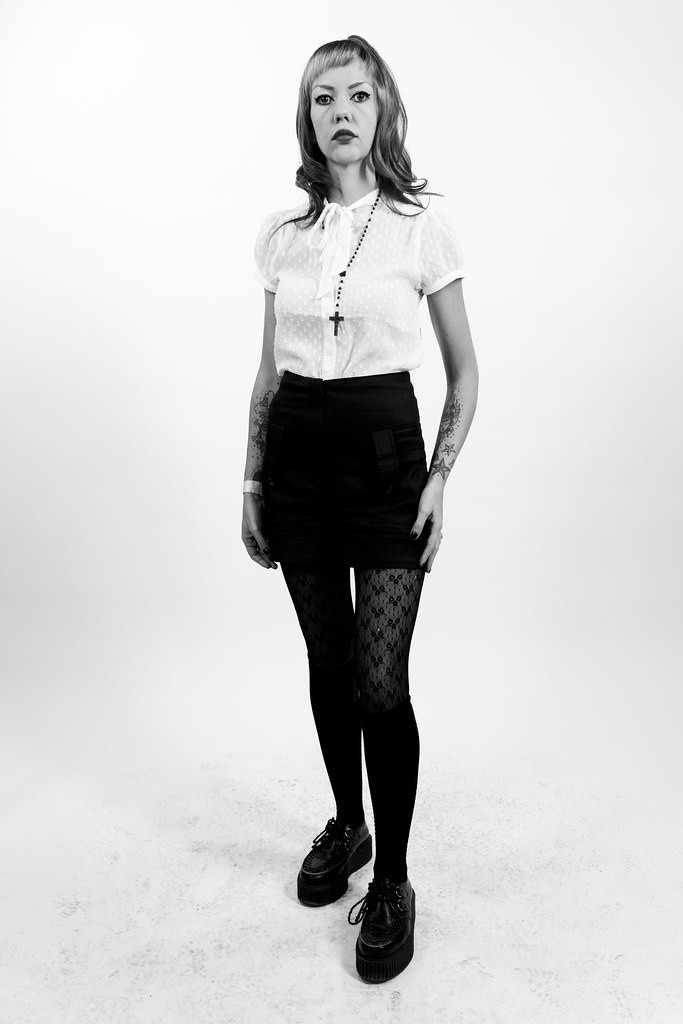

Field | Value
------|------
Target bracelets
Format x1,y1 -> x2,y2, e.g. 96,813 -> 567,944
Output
242,480 -> 263,496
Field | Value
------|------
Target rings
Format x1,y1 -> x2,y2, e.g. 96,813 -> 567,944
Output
440,533 -> 443,539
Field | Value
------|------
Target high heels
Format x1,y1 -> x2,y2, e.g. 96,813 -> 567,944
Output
296,817 -> 372,908
348,877 -> 416,985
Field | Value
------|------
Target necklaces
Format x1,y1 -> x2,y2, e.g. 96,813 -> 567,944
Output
321,189 -> 382,334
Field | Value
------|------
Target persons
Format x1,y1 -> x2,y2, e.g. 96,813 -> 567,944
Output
241,34 -> 479,983
